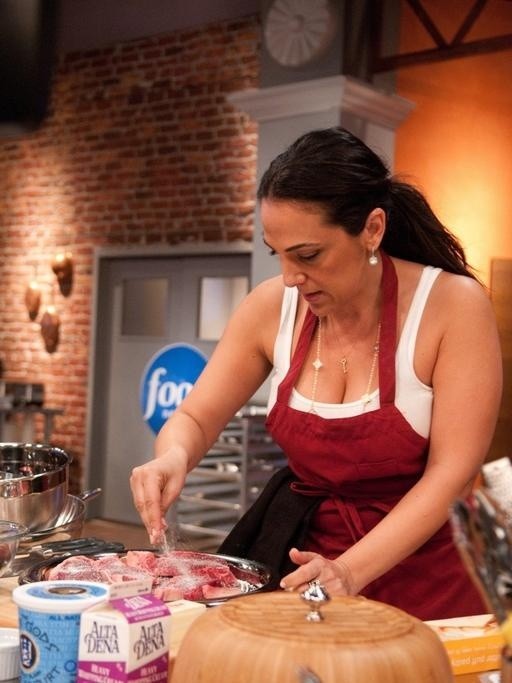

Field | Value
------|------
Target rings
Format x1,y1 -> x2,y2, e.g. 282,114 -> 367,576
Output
308,578 -> 321,588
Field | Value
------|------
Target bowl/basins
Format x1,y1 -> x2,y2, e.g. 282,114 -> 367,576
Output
0,520 -> 30,578
17,548 -> 278,608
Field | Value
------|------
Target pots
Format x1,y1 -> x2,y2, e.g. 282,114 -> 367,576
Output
1,488 -> 104,578
0,440 -> 75,530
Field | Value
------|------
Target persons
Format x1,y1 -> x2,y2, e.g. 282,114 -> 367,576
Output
130,126 -> 506,625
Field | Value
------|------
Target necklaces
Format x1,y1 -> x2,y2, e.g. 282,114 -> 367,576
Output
308,317 -> 381,415
